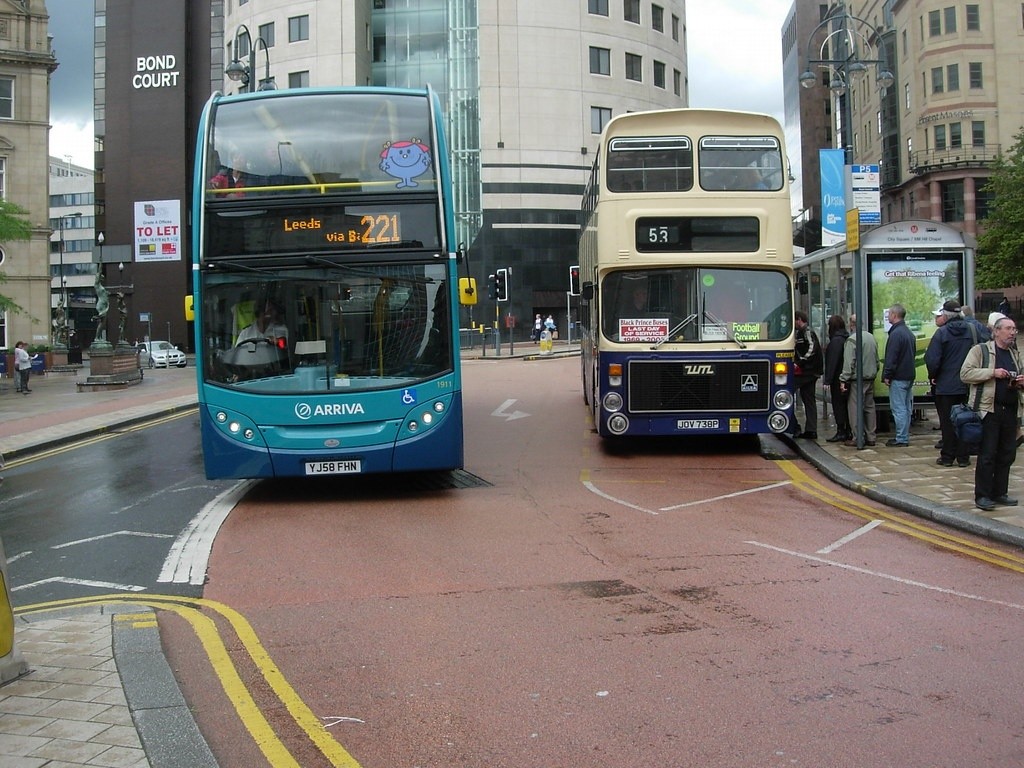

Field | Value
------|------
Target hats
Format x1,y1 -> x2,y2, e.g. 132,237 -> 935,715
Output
931,307 -> 943,316
828,316 -> 842,325
939,301 -> 961,313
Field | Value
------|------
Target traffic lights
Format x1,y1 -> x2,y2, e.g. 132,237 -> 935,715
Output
494,269 -> 507,301
570,266 -> 580,296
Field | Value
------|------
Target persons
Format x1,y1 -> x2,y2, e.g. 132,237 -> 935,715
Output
235,299 -> 289,349
823,315 -> 853,441
792,311 -> 823,439
924,301 -> 993,466
702,151 -> 769,190
707,282 -> 747,324
116,292 -> 127,342
55,298 -> 66,346
14,341 -> 32,394
532,313 -> 556,344
267,141 -> 320,195
1000,297 -> 1010,316
960,311 -> 1024,510
210,147 -> 258,198
94,257 -> 109,342
881,304 -> 916,447
838,314 -> 880,446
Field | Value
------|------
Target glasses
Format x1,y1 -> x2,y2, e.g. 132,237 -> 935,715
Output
1000,328 -> 1019,333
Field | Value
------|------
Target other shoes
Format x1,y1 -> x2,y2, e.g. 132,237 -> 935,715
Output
937,457 -> 952,466
792,424 -> 801,438
23,389 -> 32,394
886,439 -> 909,447
844,439 -> 875,446
959,459 -> 971,467
935,440 -> 942,448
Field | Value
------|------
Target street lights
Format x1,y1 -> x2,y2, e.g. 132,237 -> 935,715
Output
278,141 -> 291,174
225,24 -> 278,94
801,9 -> 895,452
59,212 -> 82,299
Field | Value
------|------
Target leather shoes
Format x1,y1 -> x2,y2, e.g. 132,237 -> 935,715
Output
975,496 -> 996,509
826,433 -> 853,442
798,432 -> 817,439
992,494 -> 1018,506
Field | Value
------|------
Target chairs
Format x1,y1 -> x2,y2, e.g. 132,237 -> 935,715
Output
230,300 -> 256,344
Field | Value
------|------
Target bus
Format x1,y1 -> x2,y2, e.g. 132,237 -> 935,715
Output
579,108 -> 808,438
183,83 -> 476,479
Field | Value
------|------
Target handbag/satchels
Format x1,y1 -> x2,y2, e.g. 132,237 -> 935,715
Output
950,404 -> 982,456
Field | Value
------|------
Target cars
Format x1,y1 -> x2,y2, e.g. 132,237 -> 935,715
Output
136,341 -> 188,369
